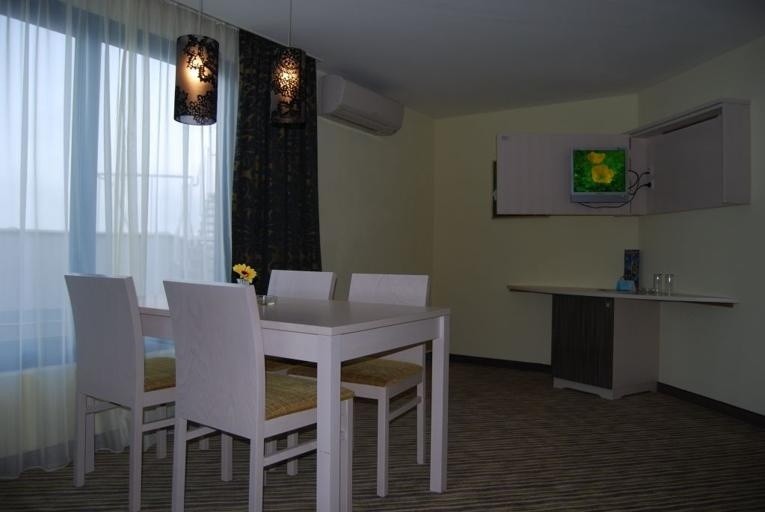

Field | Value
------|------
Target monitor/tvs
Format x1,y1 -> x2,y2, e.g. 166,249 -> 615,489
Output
567,144 -> 630,203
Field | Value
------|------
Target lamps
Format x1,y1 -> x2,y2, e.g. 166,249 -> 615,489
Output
175,0 -> 219,125
270,0 -> 307,129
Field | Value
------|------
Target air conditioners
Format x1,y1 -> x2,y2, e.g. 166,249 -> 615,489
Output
316,73 -> 404,137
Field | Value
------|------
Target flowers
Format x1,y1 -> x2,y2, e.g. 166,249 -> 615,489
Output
233,264 -> 257,283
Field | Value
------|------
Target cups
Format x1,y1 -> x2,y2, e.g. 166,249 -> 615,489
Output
653,273 -> 662,297
257,296 -> 265,306
265,295 -> 277,306
665,274 -> 675,297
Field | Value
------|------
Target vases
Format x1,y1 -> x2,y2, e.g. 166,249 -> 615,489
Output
236,278 -> 249,284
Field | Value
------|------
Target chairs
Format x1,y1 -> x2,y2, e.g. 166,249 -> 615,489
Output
162,278 -> 354,512
285,272 -> 431,498
64,272 -> 233,512
201,269 -> 337,468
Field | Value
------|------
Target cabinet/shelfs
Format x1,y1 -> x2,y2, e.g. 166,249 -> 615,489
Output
508,284 -> 738,401
495,95 -> 751,216
